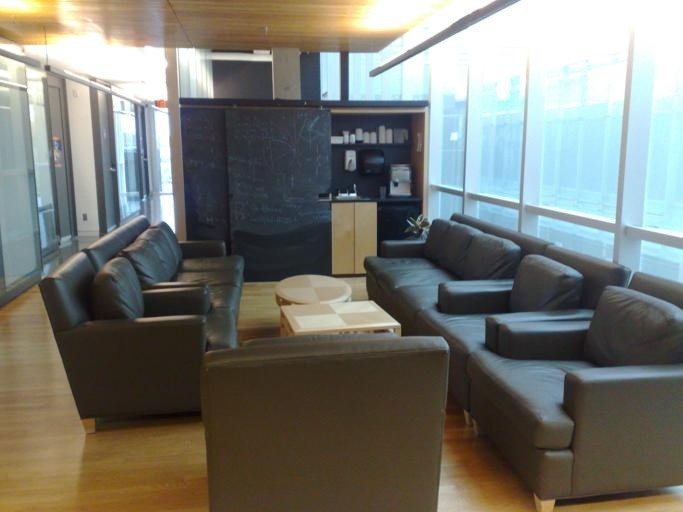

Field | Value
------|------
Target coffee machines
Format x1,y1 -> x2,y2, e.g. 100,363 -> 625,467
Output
382,163 -> 413,198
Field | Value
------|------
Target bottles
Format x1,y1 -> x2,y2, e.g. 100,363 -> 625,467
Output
343,125 -> 393,145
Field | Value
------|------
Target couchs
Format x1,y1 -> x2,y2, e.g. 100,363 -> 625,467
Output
39,214 -> 244,424
366,212 -> 683,512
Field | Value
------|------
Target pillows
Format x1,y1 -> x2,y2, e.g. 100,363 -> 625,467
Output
424,218 -> 521,281
583,285 -> 683,366
509,255 -> 585,312
122,219 -> 183,286
93,257 -> 145,319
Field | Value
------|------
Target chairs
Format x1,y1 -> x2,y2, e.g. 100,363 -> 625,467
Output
201,332 -> 450,512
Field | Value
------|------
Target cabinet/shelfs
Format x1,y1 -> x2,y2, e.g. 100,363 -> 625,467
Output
330,195 -> 377,275
331,107 -> 428,234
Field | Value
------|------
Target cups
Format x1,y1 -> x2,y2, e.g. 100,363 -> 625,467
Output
379,185 -> 386,199
